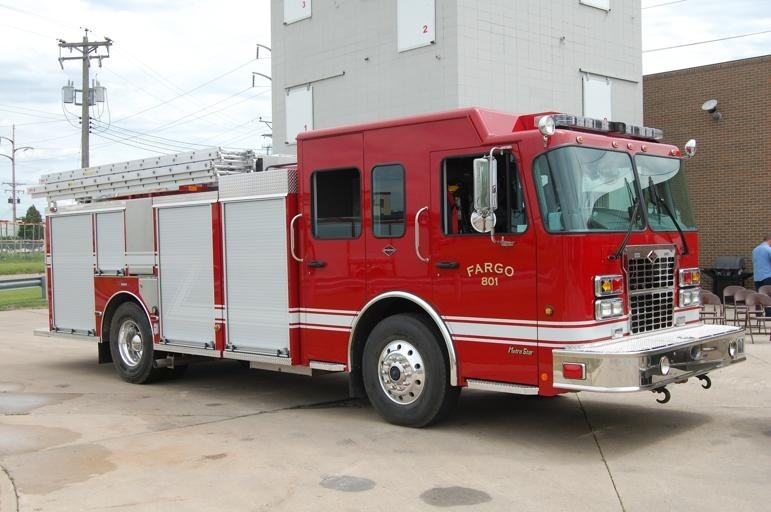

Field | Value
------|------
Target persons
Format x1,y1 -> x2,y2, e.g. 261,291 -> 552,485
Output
752,235 -> 771,321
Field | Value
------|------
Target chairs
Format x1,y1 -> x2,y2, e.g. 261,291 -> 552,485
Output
699,285 -> 771,344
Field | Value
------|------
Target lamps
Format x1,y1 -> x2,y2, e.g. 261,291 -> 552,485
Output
702,99 -> 721,120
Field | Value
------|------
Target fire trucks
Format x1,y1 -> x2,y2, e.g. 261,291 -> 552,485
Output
23,107 -> 747,428
